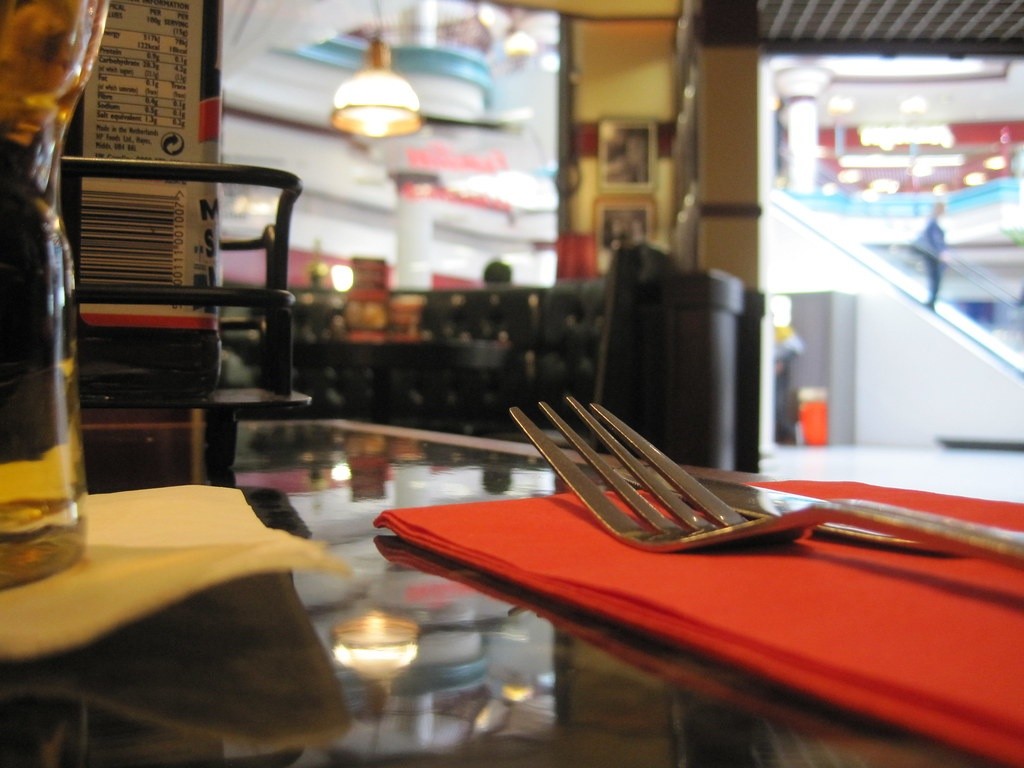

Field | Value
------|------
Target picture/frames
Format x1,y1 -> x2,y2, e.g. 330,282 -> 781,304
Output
595,115 -> 658,193
594,196 -> 657,274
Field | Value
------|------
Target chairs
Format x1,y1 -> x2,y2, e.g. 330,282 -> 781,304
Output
54,156 -> 304,413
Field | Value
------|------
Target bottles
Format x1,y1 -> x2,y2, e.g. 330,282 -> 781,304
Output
1,0 -> 106,588
63,1 -> 226,393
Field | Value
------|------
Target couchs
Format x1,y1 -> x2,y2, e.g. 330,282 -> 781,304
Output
224,160 -> 753,468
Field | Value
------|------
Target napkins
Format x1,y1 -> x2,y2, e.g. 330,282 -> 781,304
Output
372,481 -> 1024,768
0,482 -> 353,656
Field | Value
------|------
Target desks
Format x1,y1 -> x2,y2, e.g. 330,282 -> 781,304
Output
291,338 -> 448,410
0,419 -> 1024,768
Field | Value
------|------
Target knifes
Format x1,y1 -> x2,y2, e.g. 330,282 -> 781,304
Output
610,463 -> 1023,554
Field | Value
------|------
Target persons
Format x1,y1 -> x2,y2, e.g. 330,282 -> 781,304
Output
776,317 -> 803,448
928,196 -> 951,309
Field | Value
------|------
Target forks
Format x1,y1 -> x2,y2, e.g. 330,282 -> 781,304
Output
510,397 -> 1024,553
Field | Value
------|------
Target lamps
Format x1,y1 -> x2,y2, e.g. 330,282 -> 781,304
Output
332,1 -> 422,139
336,613 -> 420,684
